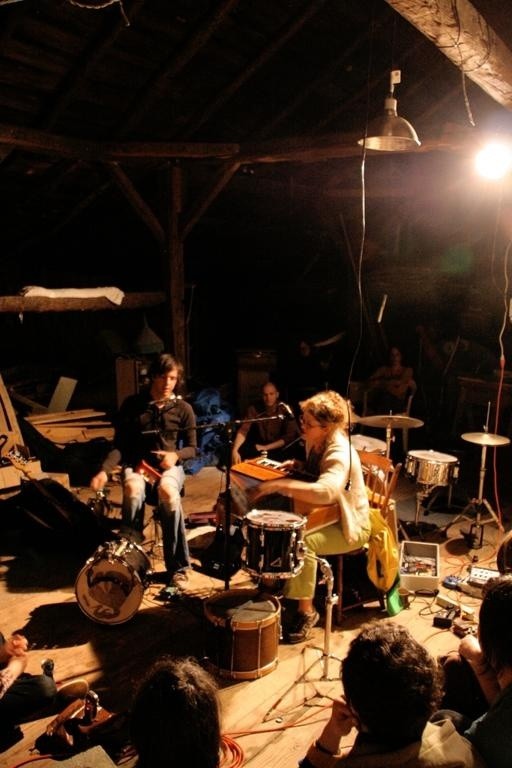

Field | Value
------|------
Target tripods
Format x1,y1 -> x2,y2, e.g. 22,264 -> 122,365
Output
264,582 -> 352,722
441,444 -> 505,547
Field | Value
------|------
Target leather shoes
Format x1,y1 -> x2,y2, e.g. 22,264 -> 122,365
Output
53,680 -> 89,703
286,609 -> 320,644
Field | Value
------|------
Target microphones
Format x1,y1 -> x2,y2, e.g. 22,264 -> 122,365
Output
281,401 -> 295,421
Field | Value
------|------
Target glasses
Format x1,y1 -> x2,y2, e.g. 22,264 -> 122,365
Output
298,414 -> 324,429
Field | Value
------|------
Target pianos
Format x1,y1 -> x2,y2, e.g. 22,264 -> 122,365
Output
231,457 -> 342,545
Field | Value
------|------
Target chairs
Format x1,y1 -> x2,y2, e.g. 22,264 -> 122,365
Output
337,449 -> 403,623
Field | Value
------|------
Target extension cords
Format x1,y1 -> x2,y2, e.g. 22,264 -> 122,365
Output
437,595 -> 476,622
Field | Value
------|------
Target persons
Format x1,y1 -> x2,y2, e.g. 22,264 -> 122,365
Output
115,353 -> 199,592
122,653 -> 222,768
437,529 -> 511,730
294,617 -> 477,768
465,576 -> 511,767
0,631 -> 90,729
299,340 -> 312,355
248,388 -> 372,645
233,380 -> 305,466
369,344 -> 419,479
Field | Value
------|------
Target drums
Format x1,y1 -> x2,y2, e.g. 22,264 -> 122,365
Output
404,449 -> 459,486
74,536 -> 154,623
350,434 -> 388,475
200,589 -> 283,679
240,509 -> 307,581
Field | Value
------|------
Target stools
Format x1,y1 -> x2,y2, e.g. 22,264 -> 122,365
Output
142,481 -> 185,562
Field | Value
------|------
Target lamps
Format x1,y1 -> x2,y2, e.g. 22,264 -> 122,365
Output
354,12 -> 422,153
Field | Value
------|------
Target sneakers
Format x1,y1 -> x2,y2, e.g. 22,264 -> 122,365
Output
171,570 -> 189,591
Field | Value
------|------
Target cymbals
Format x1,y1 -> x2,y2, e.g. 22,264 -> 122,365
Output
357,416 -> 424,429
461,433 -> 510,445
